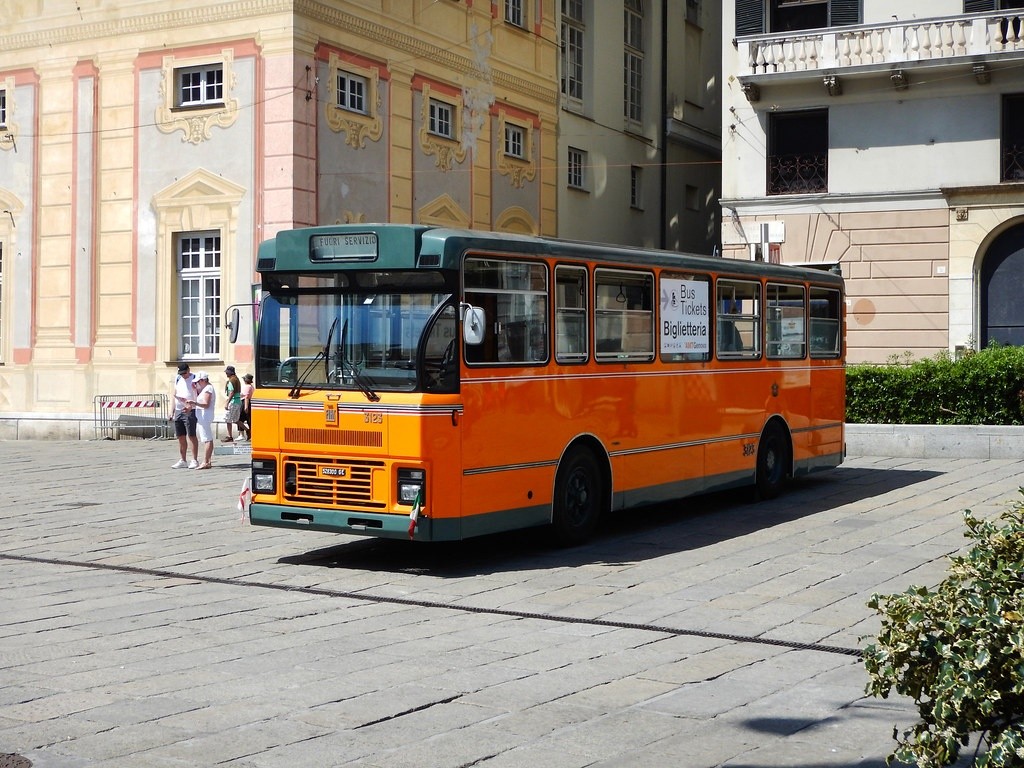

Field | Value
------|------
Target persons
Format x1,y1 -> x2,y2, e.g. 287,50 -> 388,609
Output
235,373 -> 255,440
186,371 -> 216,470
221,366 -> 251,442
717,301 -> 743,357
168,364 -> 199,469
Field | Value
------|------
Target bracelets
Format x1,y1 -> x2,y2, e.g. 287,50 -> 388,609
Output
195,402 -> 197,405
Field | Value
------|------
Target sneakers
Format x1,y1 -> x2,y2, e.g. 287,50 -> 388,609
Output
195,461 -> 212,470
188,460 -> 200,469
221,436 -> 233,442
234,435 -> 246,441
246,429 -> 251,441
171,459 -> 188,468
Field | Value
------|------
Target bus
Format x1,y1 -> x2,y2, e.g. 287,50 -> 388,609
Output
225,224 -> 846,549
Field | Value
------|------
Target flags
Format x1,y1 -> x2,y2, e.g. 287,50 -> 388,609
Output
408,489 -> 422,541
238,478 -> 249,523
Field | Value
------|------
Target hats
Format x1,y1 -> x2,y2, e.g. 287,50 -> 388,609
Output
177,362 -> 189,374
242,374 -> 254,383
224,366 -> 236,375
191,370 -> 209,383
